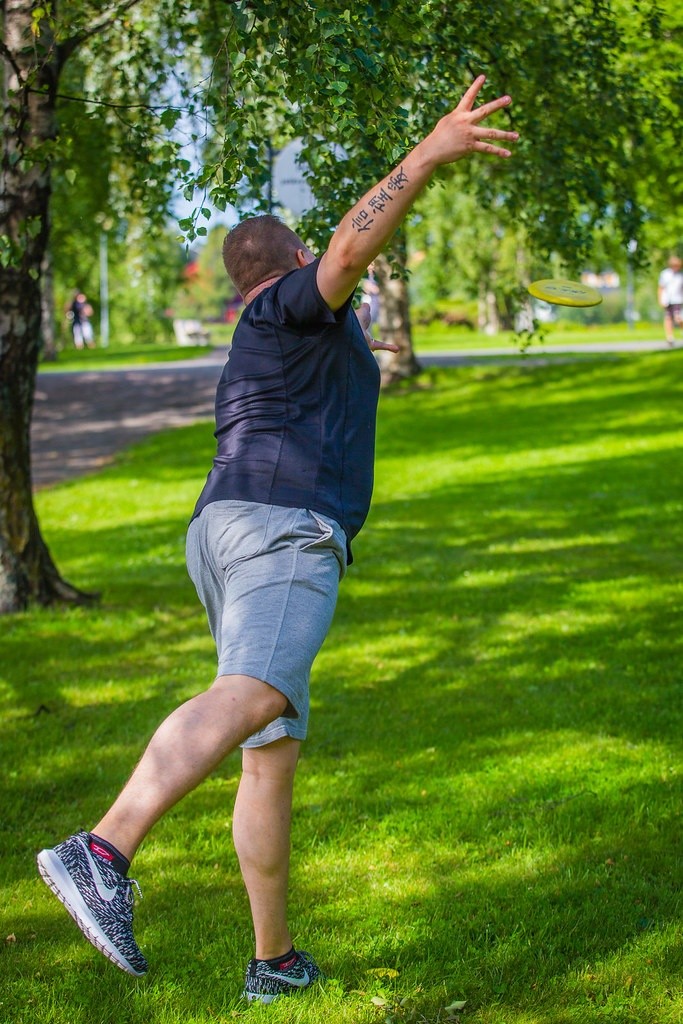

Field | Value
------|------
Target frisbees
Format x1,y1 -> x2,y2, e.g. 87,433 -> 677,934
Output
528,280 -> 603,307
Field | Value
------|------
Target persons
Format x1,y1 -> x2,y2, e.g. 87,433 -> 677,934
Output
361,262 -> 379,324
32,74 -> 519,1005
658,256 -> 683,343
65,288 -> 93,349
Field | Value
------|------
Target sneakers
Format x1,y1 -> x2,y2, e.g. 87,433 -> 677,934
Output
37,828 -> 150,978
241,950 -> 321,1003
